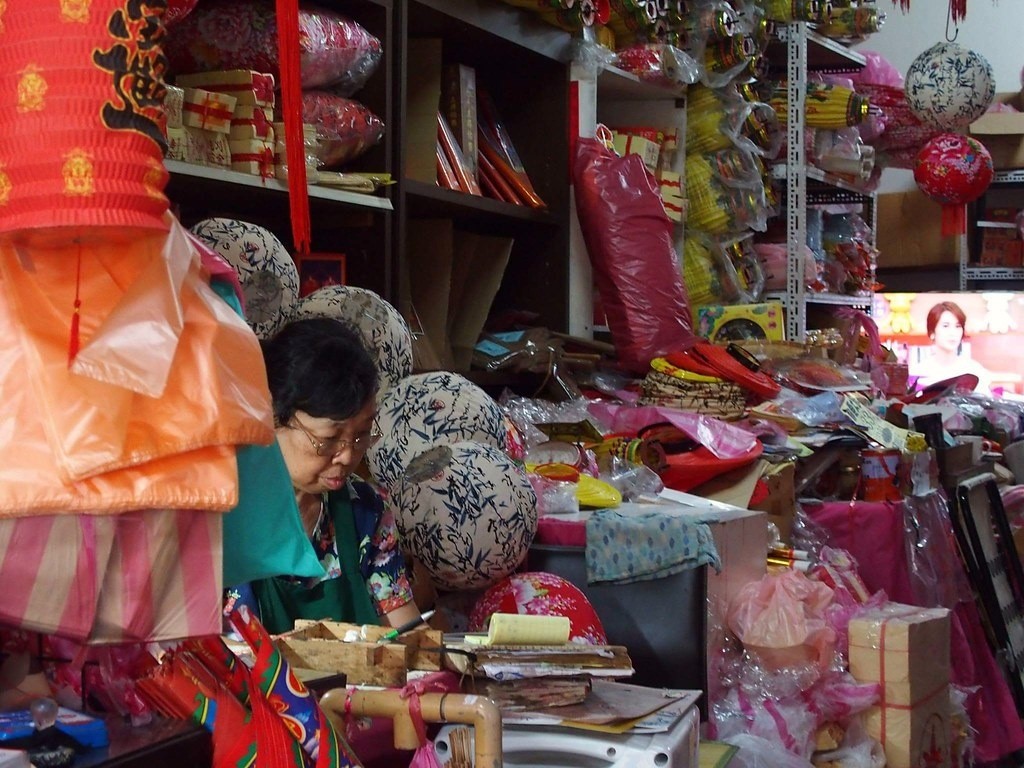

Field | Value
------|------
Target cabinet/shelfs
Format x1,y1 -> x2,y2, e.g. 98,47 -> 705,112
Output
160,0 -> 687,377
960,170 -> 1024,291
745,20 -> 876,344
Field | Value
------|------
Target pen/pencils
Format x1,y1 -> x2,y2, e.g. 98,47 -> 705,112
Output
388,609 -> 437,639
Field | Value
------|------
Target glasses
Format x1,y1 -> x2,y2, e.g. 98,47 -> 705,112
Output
292,413 -> 383,458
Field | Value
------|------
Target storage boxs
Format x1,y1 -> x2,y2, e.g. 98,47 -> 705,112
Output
960,93 -> 1024,169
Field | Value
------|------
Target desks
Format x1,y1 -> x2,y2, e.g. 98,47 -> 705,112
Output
0,664 -> 351,768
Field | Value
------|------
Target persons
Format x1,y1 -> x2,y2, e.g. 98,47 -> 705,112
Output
908,302 -> 993,401
250,322 -> 434,635
